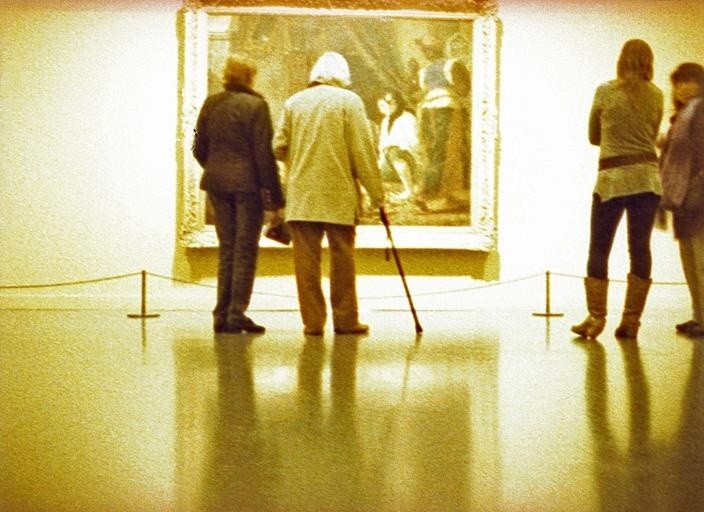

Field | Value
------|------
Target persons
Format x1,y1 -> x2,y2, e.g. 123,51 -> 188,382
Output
570,40 -> 664,339
191,52 -> 287,334
363,32 -> 470,213
270,51 -> 386,334
653,61 -> 704,337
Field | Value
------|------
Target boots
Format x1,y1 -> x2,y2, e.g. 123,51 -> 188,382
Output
572,277 -> 609,340
615,273 -> 653,339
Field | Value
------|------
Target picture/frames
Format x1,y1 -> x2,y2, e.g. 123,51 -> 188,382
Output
177,5 -> 501,254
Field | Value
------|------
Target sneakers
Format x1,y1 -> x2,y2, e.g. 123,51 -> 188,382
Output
676,321 -> 703,335
305,323 -> 367,334
214,323 -> 263,333
